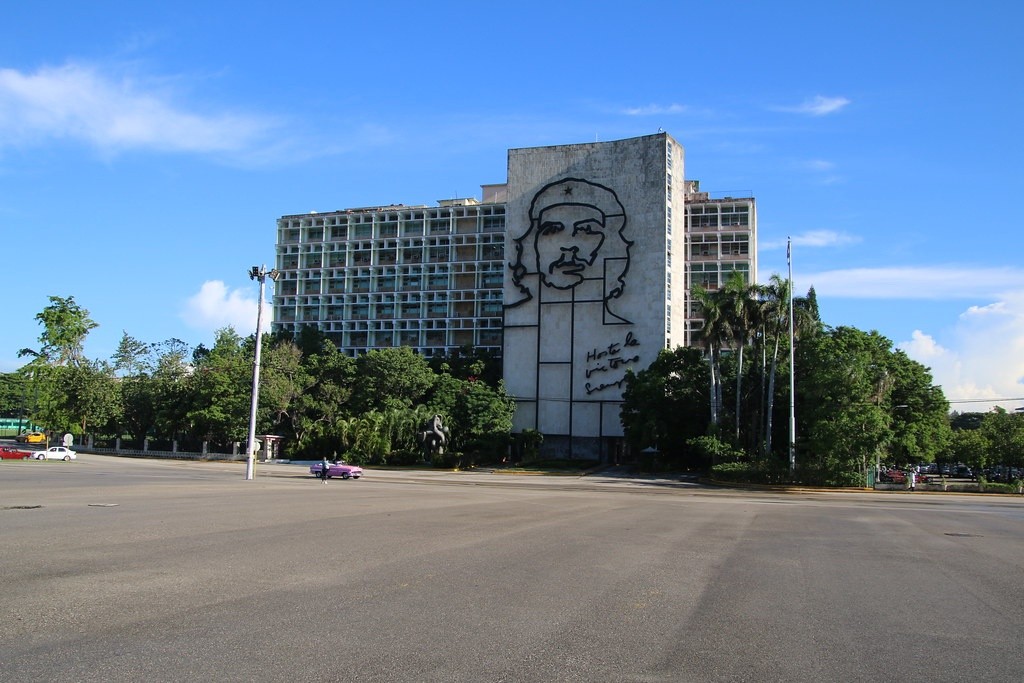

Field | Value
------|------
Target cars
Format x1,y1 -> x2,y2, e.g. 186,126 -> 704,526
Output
921,462 -> 1019,482
310,461 -> 363,480
0,445 -> 77,461
15,431 -> 52,443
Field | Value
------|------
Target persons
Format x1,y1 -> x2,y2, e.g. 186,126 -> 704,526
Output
320,457 -> 330,484
502,457 -> 507,468
905,467 -> 916,492
332,451 -> 337,464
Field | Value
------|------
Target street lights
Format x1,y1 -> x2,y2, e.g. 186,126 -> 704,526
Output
876,405 -> 908,484
244,264 -> 281,479
